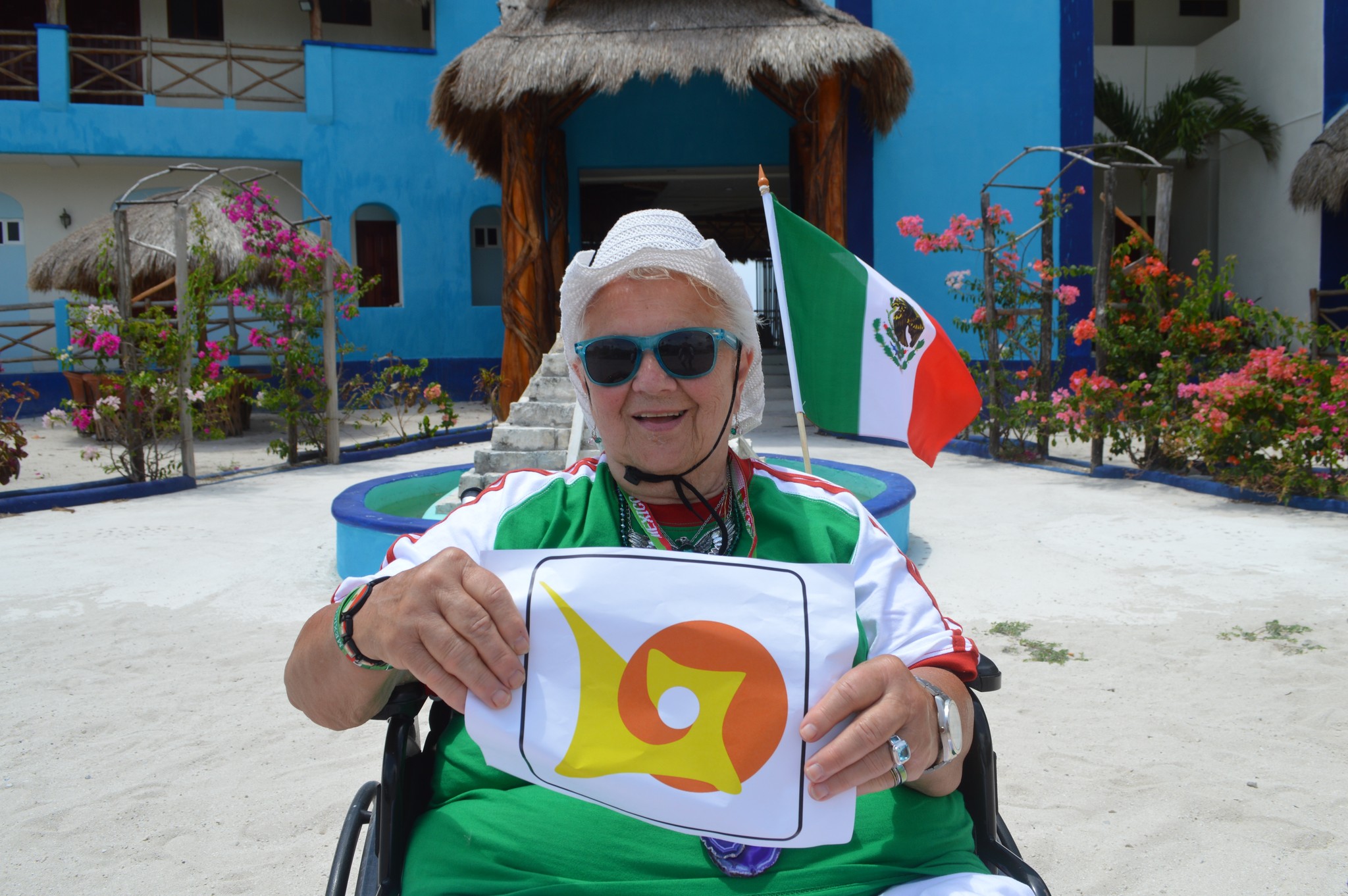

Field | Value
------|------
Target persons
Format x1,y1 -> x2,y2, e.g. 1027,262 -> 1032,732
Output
283,212 -> 1035,895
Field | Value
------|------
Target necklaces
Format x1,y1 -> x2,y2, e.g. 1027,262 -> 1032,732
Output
613,462 -> 742,556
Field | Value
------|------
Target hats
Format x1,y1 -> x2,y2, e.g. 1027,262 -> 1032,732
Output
559,209 -> 766,453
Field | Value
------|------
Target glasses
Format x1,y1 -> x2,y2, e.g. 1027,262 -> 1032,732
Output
574,327 -> 741,387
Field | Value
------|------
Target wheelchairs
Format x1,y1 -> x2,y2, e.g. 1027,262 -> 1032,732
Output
327,654 -> 1050,896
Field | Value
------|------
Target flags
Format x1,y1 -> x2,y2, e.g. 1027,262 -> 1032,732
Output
760,192 -> 985,470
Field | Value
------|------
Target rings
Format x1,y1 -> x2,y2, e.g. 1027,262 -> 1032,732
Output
889,765 -> 907,788
887,732 -> 911,765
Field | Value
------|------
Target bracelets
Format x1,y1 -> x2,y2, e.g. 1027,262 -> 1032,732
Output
332,575 -> 394,670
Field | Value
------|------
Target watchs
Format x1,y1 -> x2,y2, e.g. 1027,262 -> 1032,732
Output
914,675 -> 963,776
